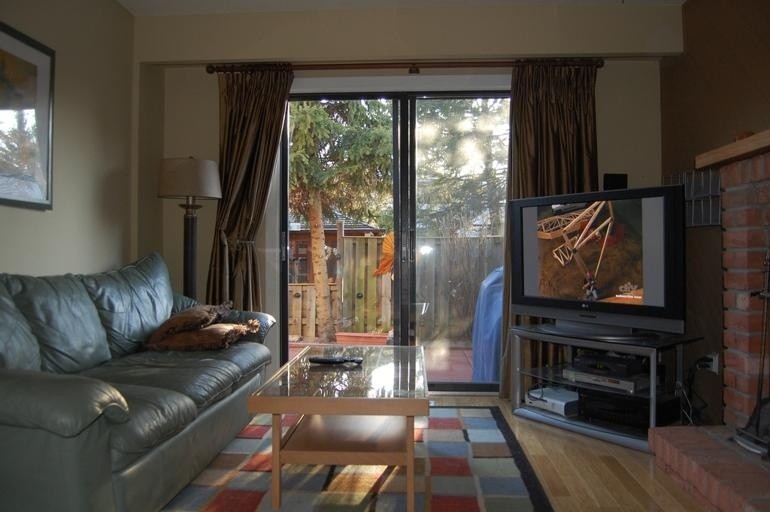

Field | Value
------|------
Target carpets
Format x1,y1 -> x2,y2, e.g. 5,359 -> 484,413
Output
156,406 -> 557,512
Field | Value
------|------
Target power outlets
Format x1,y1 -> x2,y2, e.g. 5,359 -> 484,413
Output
704,354 -> 719,374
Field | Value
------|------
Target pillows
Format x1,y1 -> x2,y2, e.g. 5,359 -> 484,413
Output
0,273 -> 113,374
0,278 -> 42,373
146,300 -> 233,343
77,252 -> 174,360
159,318 -> 260,351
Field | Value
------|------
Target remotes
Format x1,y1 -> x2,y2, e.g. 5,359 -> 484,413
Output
308,356 -> 363,365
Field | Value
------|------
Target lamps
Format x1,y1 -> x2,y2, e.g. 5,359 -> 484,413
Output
415,237 -> 434,258
156,157 -> 222,301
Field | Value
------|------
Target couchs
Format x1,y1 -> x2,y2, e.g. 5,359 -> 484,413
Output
0,249 -> 276,512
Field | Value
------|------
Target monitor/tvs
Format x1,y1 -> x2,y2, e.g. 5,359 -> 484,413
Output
509,186 -> 686,342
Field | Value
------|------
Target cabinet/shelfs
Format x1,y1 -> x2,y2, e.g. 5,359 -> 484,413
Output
507,324 -> 704,456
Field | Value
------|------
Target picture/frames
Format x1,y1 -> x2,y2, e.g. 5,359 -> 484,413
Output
0,22 -> 55,210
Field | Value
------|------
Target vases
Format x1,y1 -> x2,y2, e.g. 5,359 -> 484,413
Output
334,331 -> 389,344
